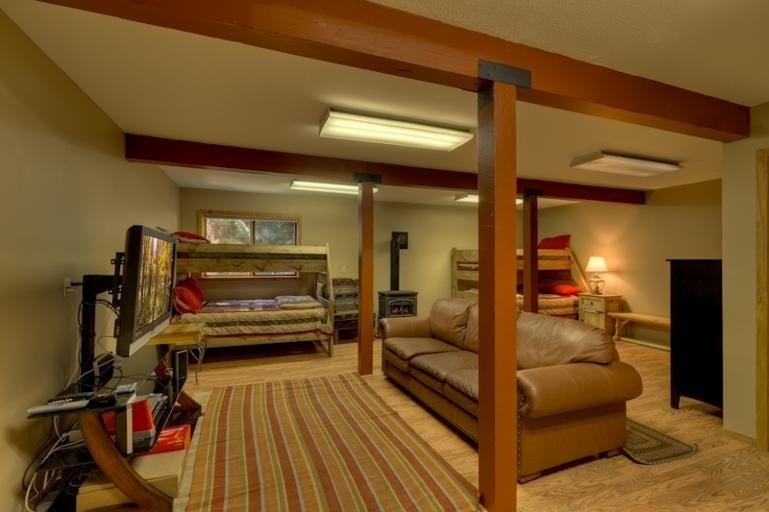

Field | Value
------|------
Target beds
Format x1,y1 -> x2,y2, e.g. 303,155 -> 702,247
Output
451,246 -> 588,320
156,243 -> 335,359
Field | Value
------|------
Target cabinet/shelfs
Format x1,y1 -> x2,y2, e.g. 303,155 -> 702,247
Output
330,277 -> 360,344
665,258 -> 724,411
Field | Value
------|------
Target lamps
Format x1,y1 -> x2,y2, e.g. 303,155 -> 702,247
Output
568,149 -> 686,178
584,255 -> 608,294
317,106 -> 475,153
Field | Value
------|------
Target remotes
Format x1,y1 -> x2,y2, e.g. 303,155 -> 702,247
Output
47,392 -> 98,398
26,399 -> 89,416
47,395 -> 115,407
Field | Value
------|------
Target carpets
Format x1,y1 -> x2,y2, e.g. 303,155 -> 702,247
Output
623,414 -> 699,466
173,370 -> 485,512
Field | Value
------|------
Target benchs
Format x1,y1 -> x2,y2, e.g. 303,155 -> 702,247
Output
606,311 -> 671,342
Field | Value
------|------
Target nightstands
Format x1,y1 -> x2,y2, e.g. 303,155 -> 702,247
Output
577,294 -> 623,335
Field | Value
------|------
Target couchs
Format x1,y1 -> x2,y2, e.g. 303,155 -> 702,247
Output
376,296 -> 644,485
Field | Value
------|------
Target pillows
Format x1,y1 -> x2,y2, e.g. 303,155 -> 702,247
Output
538,278 -> 582,297
539,233 -> 572,248
170,230 -> 212,244
171,274 -> 210,315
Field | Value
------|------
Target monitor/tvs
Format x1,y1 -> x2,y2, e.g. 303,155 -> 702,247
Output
115,224 -> 178,358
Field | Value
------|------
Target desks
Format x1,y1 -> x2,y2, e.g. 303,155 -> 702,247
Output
24,342 -> 202,511
146,321 -> 209,386
